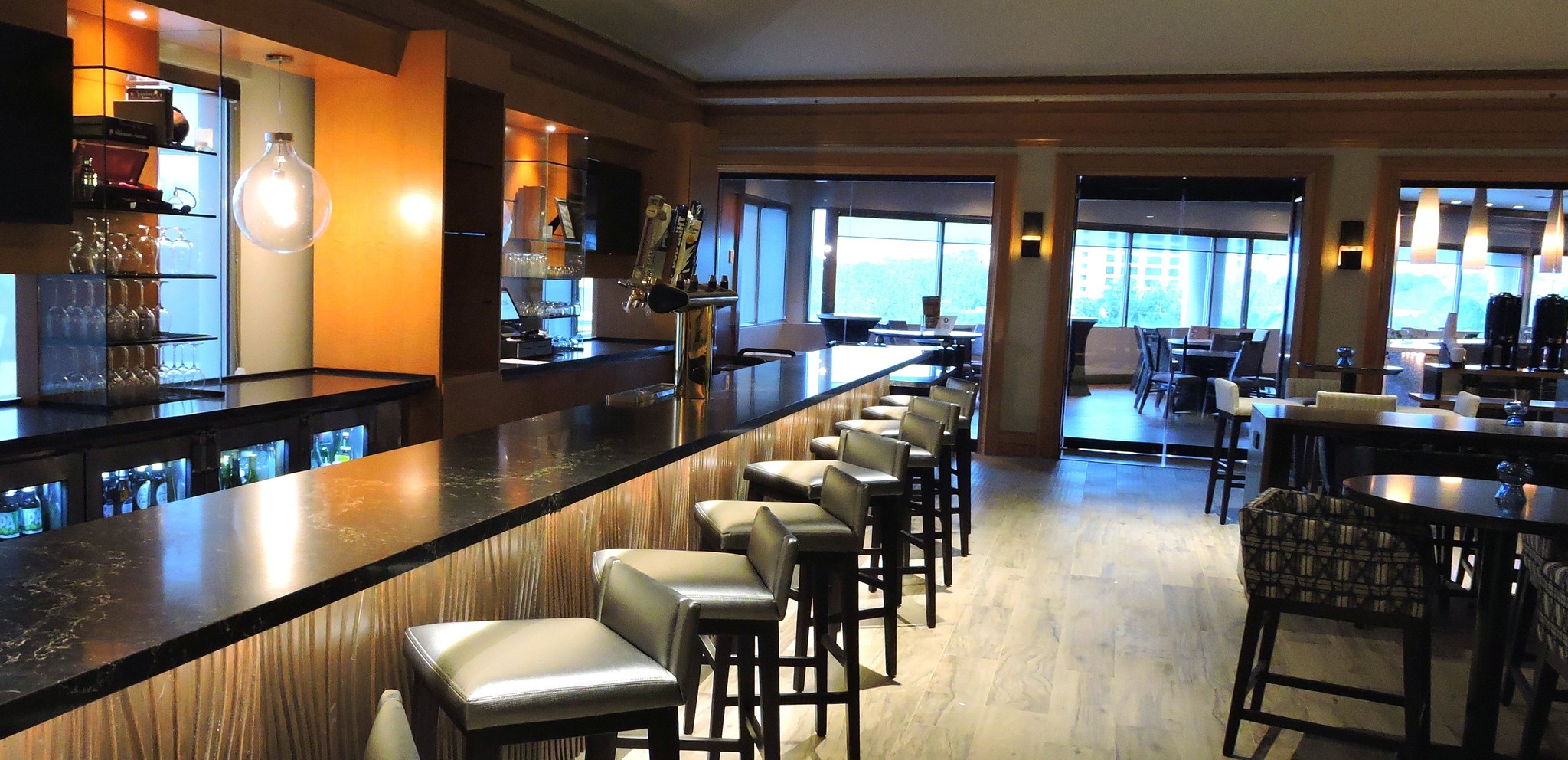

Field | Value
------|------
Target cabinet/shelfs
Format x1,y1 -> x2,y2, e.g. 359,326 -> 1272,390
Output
0,403 -> 403,544
500,159 -> 584,353
0,126 -> 224,412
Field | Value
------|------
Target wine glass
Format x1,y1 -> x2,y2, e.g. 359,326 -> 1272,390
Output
43,216 -> 206,407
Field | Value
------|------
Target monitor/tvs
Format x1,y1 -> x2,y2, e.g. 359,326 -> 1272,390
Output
0,21 -> 75,225
583,157 -> 642,256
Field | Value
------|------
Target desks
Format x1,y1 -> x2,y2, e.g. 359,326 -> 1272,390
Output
867,328 -> 984,367
1335,473 -> 1568,759
1165,337 -> 1212,349
1171,347 -> 1239,412
816,313 -> 880,349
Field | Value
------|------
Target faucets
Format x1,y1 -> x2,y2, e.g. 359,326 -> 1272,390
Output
620,290 -> 653,316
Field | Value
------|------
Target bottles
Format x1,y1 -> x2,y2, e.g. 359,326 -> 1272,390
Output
310,431 -> 353,467
220,447 -> 277,491
101,462 -> 177,518
74,155 -> 98,200
1,486 -> 42,541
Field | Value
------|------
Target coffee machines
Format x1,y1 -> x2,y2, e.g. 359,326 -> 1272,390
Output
1529,294 -> 1568,372
1482,291 -> 1522,370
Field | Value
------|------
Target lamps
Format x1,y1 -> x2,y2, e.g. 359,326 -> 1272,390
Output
232,54 -> 330,252
1393,183 -> 1565,275
1338,221 -> 1363,269
1020,212 -> 1043,259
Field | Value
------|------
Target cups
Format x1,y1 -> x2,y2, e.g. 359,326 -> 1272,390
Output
504,253 -> 580,353
1539,347 -> 1550,368
1492,345 -> 1503,366
1444,312 -> 1467,363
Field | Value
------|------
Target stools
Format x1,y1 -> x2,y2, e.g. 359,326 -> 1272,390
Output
361,378 -> 1398,760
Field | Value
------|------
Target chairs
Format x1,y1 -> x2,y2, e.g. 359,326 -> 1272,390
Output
879,318 -> 1568,759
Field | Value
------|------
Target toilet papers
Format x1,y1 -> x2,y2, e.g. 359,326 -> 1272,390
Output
1443,312 -> 1457,345
